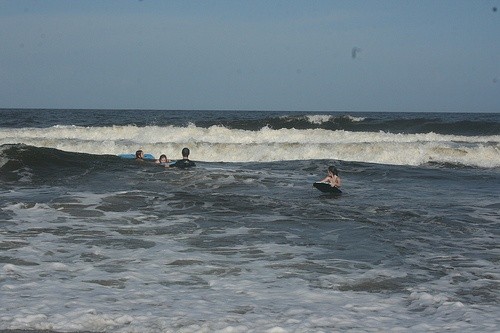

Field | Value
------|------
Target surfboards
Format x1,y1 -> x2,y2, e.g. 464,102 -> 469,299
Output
312,181 -> 343,192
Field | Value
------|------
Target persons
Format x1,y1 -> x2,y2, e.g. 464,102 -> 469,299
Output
321,166 -> 341,188
155,154 -> 170,163
136,150 -> 144,161
166,147 -> 196,168
